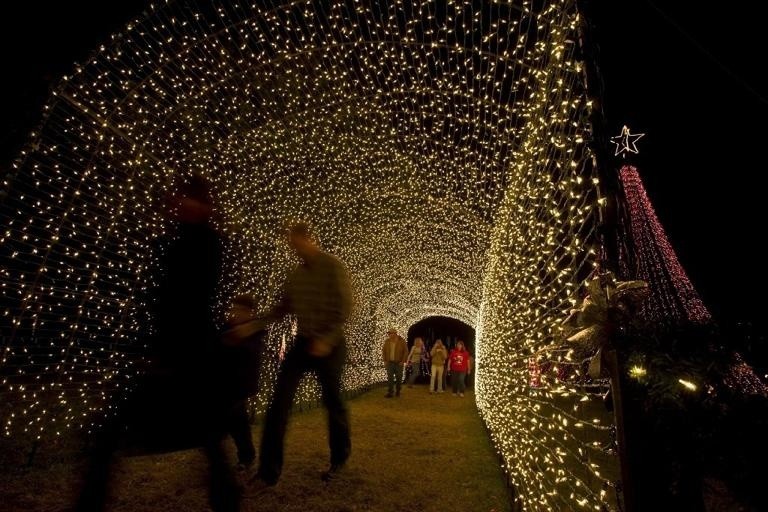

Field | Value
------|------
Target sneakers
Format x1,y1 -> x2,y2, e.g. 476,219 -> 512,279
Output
384,393 -> 393,397
242,474 -> 280,498
397,392 -> 399,396
452,393 -> 464,398
322,461 -> 345,480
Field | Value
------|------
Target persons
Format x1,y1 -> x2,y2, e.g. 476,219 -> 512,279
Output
211,291 -> 267,474
447,340 -> 471,398
406,337 -> 425,389
225,222 -> 356,497
429,338 -> 449,394
65,170 -> 249,511
382,327 -> 410,398
